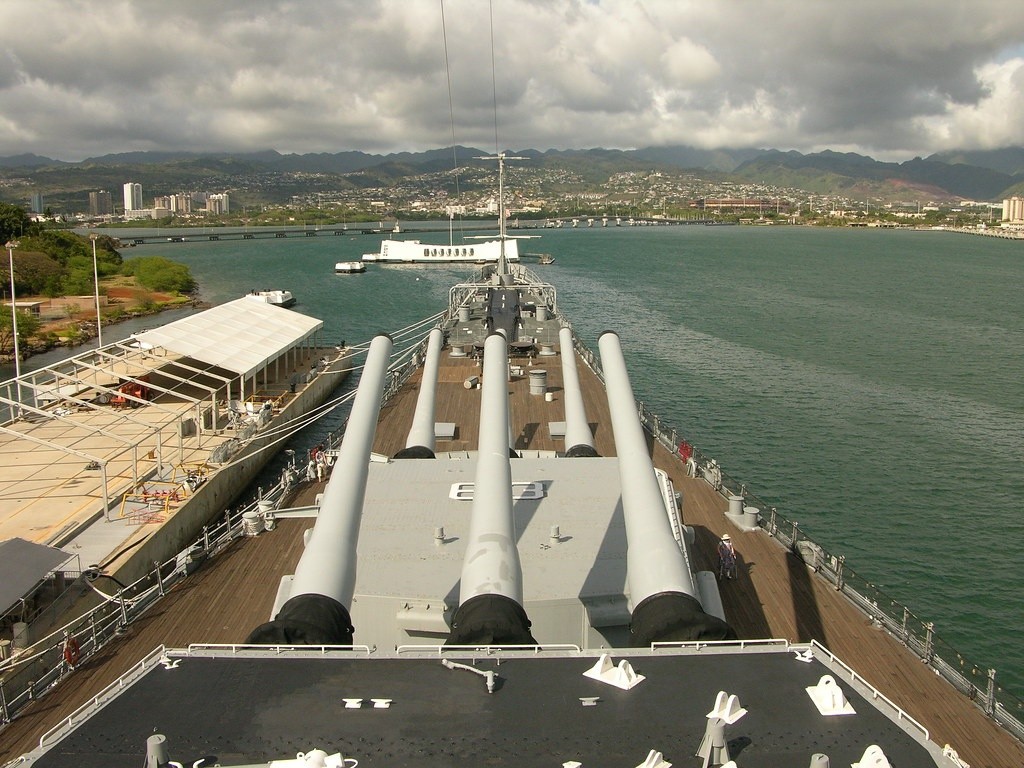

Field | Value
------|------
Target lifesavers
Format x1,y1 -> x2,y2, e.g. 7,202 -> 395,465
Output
65,638 -> 79,666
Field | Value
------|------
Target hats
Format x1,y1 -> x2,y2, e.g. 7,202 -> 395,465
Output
721,534 -> 731,540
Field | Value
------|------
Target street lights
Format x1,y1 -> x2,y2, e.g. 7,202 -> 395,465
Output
5,240 -> 25,418
89,233 -> 102,348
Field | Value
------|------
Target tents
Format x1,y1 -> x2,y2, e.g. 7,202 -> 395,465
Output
1,537 -> 81,627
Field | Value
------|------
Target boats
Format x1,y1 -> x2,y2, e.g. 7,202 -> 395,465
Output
247,288 -> 296,309
335,261 -> 367,274
362,214 -> 521,265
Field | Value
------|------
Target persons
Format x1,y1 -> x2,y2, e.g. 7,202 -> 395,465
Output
309,443 -> 328,483
719,535 -> 735,581
